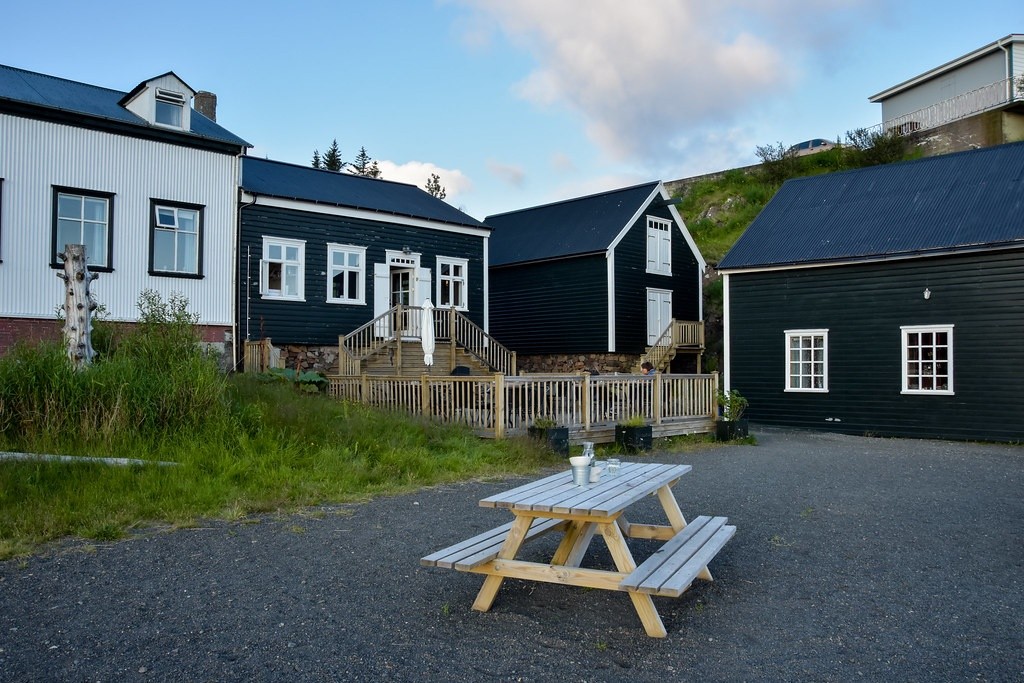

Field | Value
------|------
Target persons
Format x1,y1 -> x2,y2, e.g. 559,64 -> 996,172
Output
575,360 -> 617,418
641,362 -> 658,375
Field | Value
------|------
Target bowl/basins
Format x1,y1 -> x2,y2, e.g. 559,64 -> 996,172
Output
569,456 -> 591,466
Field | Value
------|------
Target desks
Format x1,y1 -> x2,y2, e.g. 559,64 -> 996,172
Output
471,461 -> 714,638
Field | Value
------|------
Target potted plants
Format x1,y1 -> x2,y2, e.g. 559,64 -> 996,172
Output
615,417 -> 653,454
716,390 -> 750,440
529,419 -> 570,459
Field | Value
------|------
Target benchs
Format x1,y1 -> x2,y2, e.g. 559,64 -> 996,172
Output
619,515 -> 738,638
420,516 -> 570,611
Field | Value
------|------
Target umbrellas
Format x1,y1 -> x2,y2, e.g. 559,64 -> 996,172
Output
421,297 -> 435,375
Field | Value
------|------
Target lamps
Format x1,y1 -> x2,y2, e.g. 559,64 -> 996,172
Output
402,246 -> 413,255
923,288 -> 932,299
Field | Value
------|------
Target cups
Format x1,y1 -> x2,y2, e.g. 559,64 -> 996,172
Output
607,458 -> 619,477
571,465 -> 591,486
589,467 -> 601,482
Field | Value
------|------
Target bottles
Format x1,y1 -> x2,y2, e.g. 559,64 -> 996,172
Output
582,441 -> 595,467
